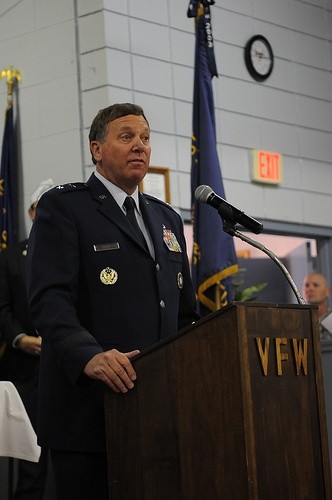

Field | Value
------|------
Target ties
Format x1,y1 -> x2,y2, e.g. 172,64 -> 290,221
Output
122,196 -> 149,255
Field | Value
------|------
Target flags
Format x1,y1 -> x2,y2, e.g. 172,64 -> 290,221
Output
0,78 -> 19,381
187,0 -> 239,320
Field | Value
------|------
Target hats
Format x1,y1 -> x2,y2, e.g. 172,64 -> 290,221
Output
27,178 -> 55,209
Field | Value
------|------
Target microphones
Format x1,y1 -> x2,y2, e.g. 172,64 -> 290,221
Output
195,184 -> 264,235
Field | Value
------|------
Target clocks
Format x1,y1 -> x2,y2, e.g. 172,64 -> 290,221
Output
244,35 -> 274,82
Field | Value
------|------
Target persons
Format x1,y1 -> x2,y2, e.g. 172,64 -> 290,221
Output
7,201 -> 41,409
21,103 -> 195,500
303,273 -> 332,464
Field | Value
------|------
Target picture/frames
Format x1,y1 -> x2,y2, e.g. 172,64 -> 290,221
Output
138,167 -> 171,203
